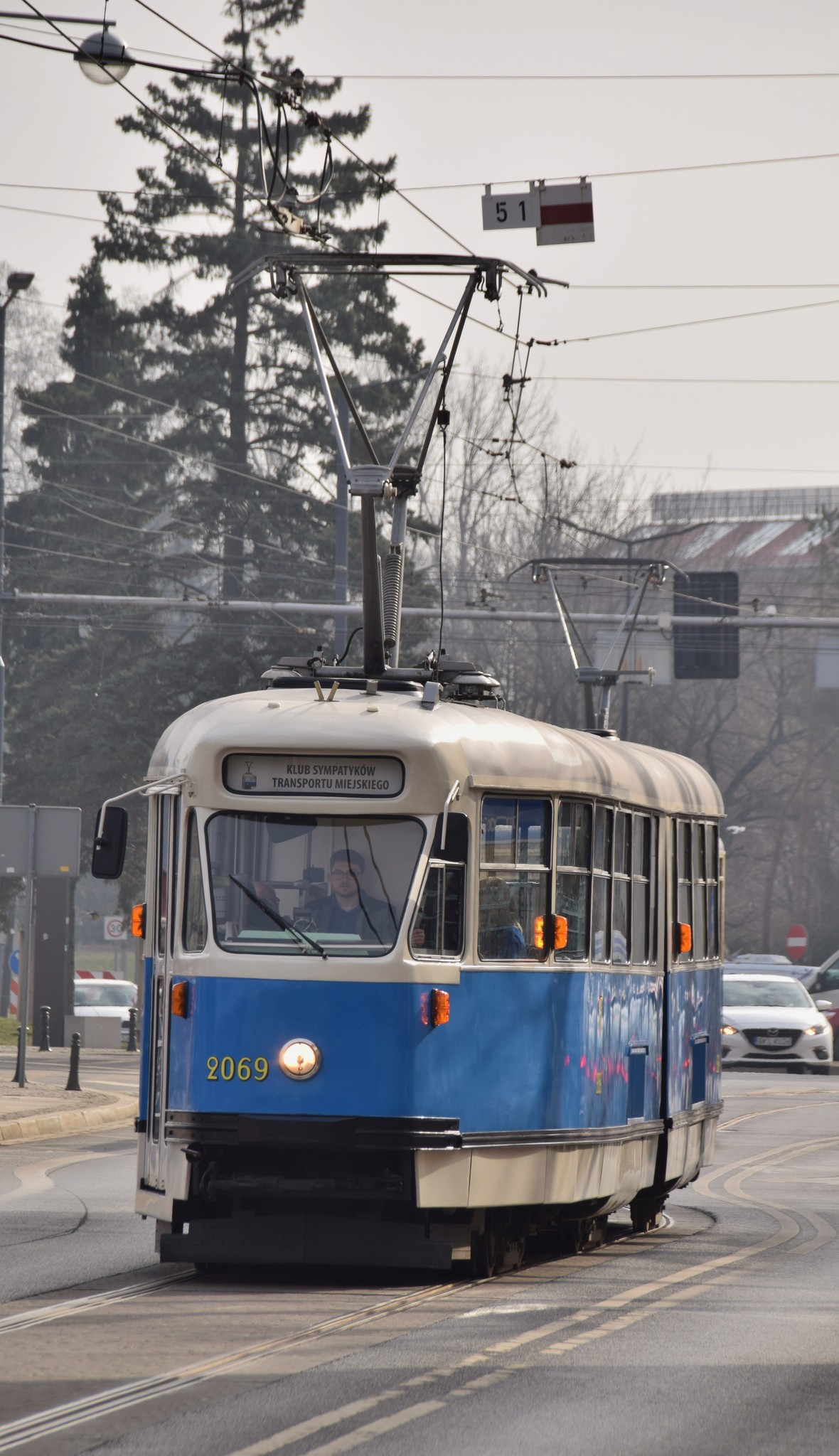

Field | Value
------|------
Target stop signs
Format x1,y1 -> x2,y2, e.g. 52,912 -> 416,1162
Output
785,924 -> 807,959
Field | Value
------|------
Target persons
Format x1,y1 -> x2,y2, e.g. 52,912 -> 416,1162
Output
253,881 -> 294,932
479,877 -> 527,959
307,849 -> 425,945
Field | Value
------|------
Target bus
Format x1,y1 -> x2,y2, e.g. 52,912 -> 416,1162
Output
89,264 -> 728,1271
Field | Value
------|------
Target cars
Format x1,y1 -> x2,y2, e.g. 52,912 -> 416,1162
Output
802,949 -> 839,1048
73,979 -> 138,1022
712,974 -> 835,1075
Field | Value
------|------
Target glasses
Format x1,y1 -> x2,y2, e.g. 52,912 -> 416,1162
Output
272,896 -> 280,906
331,870 -> 360,880
